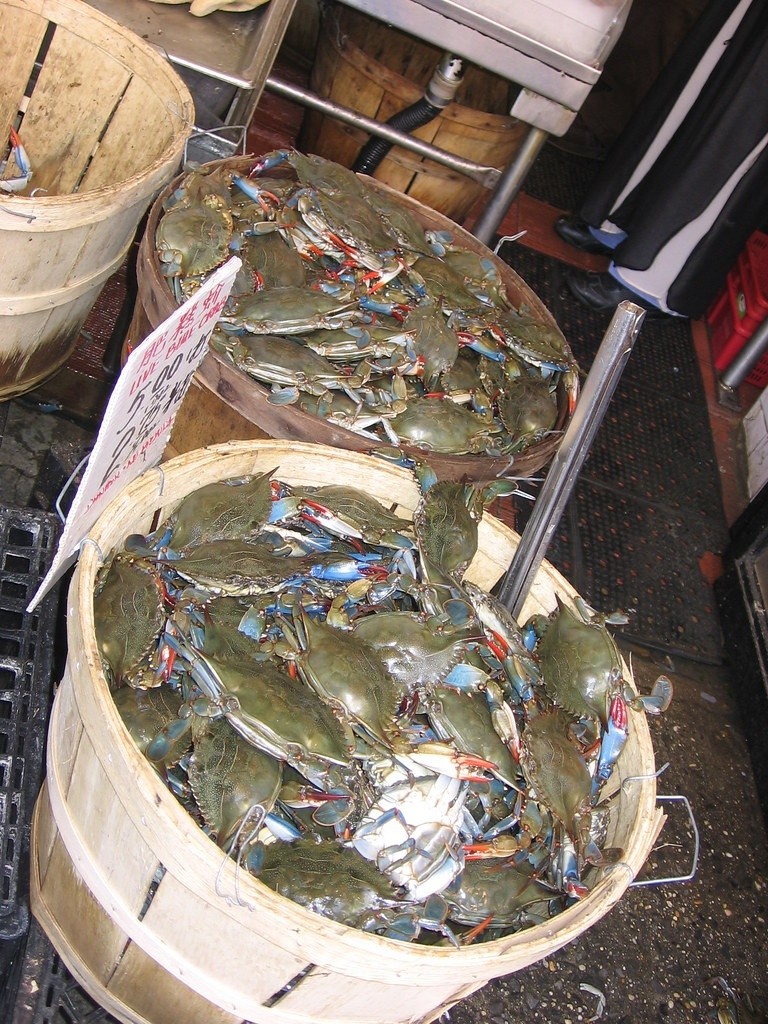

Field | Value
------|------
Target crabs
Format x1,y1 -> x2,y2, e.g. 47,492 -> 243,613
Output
0,124 -> 674,948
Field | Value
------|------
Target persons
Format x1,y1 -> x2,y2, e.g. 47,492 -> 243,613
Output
554,0 -> 767,321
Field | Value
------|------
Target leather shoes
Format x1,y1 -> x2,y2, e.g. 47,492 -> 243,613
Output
554,213 -> 621,257
563,262 -> 673,323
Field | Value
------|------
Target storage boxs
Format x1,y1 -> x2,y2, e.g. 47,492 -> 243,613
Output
0,501 -> 121,1024
704,230 -> 768,388
